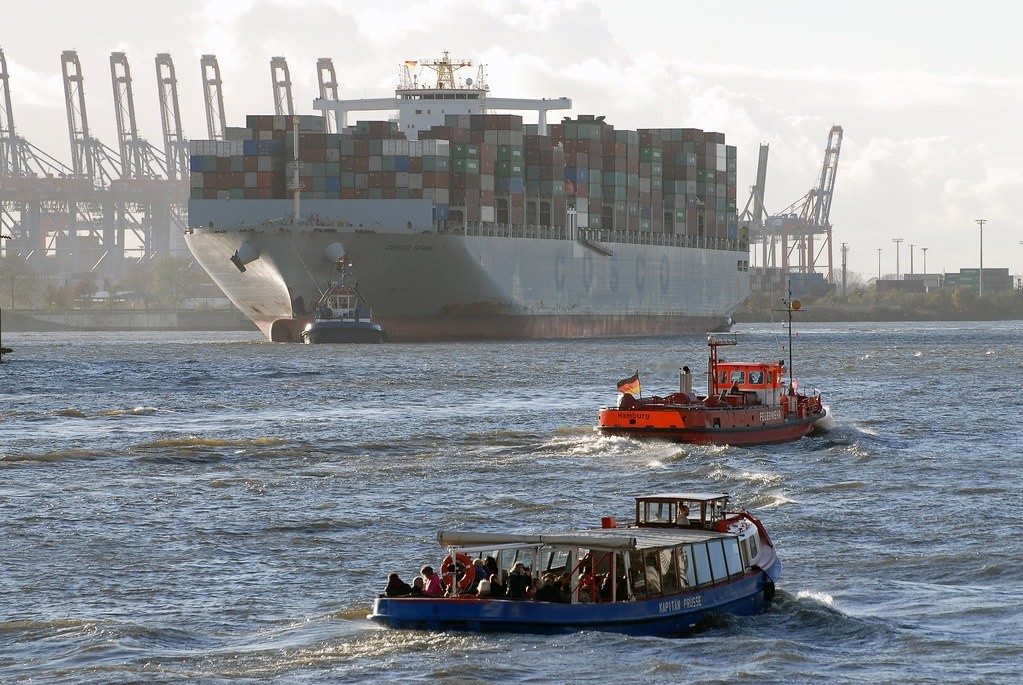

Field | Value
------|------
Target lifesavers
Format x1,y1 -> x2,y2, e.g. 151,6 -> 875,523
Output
443,553 -> 475,588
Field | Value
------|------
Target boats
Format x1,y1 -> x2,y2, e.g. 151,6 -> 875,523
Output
592,277 -> 827,449
300,253 -> 386,345
365,490 -> 783,637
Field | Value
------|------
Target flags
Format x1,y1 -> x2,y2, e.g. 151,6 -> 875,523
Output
405,61 -> 417,68
617,375 -> 640,395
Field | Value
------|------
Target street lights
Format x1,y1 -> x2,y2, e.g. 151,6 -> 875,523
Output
877,249 -> 883,280
892,239 -> 903,280
975,218 -> 987,295
922,247 -> 927,284
908,245 -> 915,273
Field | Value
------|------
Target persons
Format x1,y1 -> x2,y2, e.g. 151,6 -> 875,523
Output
731,381 -> 739,395
473,559 -> 486,580
672,505 -> 690,524
421,565 -> 443,594
435,556 -> 625,603
385,573 -> 411,596
410,576 -> 424,596
749,373 -> 753,383
758,372 -> 768,384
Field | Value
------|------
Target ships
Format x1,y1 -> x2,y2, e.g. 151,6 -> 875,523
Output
181,50 -> 748,336
